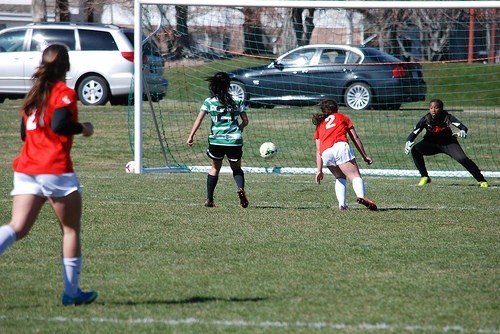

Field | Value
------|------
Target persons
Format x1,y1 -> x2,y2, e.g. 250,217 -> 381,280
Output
0,44 -> 98,305
187,72 -> 248,208
405,99 -> 488,188
312,99 -> 377,211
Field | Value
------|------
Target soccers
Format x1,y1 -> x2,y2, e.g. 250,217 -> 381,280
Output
125,160 -> 134,175
259,142 -> 278,158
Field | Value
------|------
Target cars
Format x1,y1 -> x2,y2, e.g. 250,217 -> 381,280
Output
226,44 -> 427,112
0,23 -> 169,108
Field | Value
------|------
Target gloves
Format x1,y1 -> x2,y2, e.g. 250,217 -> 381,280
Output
452,130 -> 467,138
404,141 -> 415,155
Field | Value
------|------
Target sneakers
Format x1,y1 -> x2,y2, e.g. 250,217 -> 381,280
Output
418,176 -> 431,186
62,288 -> 98,305
236,187 -> 248,208
357,197 -> 377,211
205,200 -> 216,207
480,181 -> 488,188
